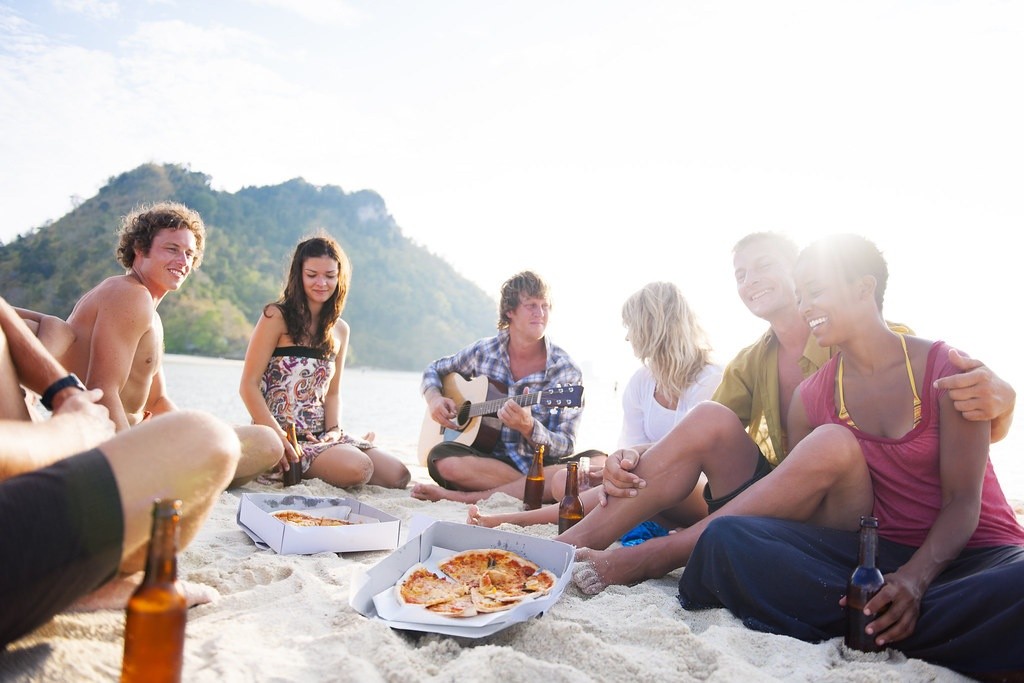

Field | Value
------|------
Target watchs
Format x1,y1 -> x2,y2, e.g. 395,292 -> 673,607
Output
41,371 -> 87,412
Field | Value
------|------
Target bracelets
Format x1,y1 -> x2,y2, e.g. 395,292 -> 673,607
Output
326,425 -> 344,442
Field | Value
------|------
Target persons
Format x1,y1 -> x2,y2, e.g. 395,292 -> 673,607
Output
467,281 -> 725,528
1,297 -> 240,652
553,234 -> 1015,595
55,201 -> 286,494
239,234 -> 410,489
678,239 -> 1024,683
410,270 -> 608,504
11,306 -> 75,360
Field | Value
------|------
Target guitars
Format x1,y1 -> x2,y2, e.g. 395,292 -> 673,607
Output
417,372 -> 585,467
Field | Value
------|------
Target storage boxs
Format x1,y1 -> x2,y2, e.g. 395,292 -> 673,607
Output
239,493 -> 577,636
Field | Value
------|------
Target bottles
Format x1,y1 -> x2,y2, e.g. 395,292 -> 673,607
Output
522,444 -> 544,511
281,422 -> 303,490
119,496 -> 189,683
843,513 -> 886,657
558,460 -> 585,534
